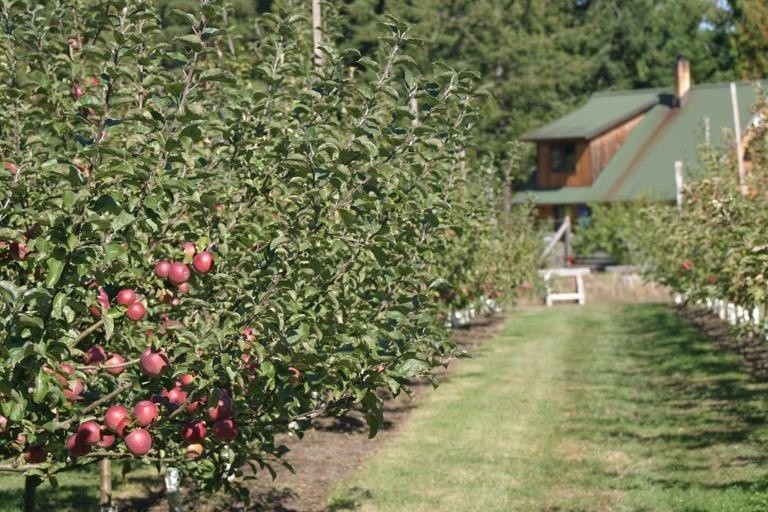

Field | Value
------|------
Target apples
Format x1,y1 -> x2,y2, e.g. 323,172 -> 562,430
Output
681,262 -> 689,270
0,38 -> 301,462
708,275 -> 715,283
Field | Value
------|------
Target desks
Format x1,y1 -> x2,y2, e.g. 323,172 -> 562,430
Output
537,265 -> 590,306
